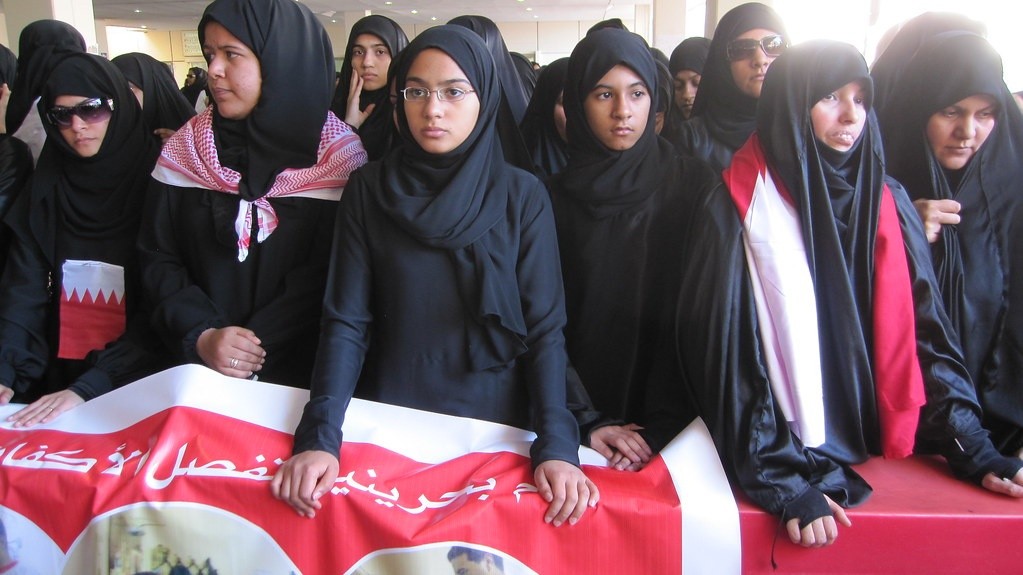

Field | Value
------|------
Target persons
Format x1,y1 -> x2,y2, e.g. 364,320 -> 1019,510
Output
138,0 -> 370,392
645,35 -> 712,145
673,0 -> 793,174
666,39 -> 1023,549
540,23 -> 720,473
0,20 -> 215,428
446,13 -> 571,183
269,23 -> 599,529
871,11 -> 1022,499
330,12 -> 411,169
447,546 -> 508,575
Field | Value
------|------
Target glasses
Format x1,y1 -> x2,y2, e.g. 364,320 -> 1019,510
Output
400,87 -> 476,102
726,33 -> 788,62
46,97 -> 115,130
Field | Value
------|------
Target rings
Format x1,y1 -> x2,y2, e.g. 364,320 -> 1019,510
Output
230,359 -> 239,368
47,406 -> 53,411
